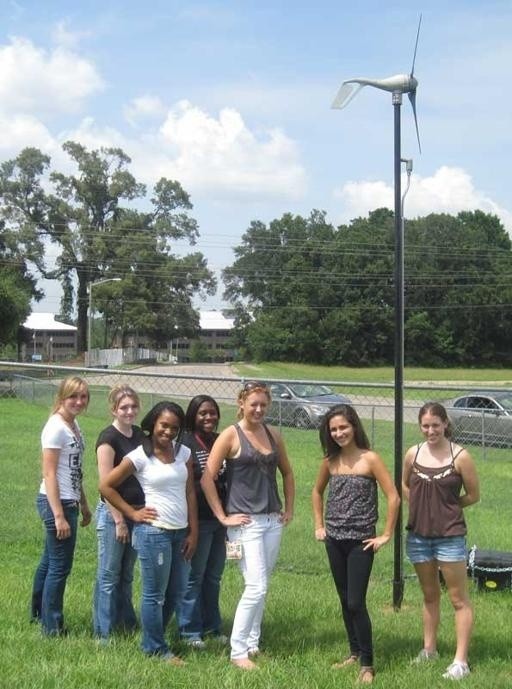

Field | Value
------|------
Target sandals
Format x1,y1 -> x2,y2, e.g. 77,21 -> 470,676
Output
354,665 -> 375,686
331,655 -> 358,671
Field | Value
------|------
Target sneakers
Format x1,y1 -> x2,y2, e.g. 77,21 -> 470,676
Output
214,635 -> 229,646
187,640 -> 206,649
441,661 -> 471,680
164,656 -> 189,668
412,649 -> 438,664
233,658 -> 260,672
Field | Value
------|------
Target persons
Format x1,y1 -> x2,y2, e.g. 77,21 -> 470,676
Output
176,393 -> 229,650
97,400 -> 200,670
92,381 -> 148,647
29,375 -> 91,641
199,377 -> 298,674
309,402 -> 401,688
399,401 -> 481,681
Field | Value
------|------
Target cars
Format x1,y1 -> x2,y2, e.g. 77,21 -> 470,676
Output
261,381 -> 354,430
434,389 -> 512,448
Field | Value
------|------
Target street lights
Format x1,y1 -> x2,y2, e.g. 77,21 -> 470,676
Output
87,277 -> 123,371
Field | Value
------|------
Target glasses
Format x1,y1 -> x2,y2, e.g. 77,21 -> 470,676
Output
244,381 -> 266,391
